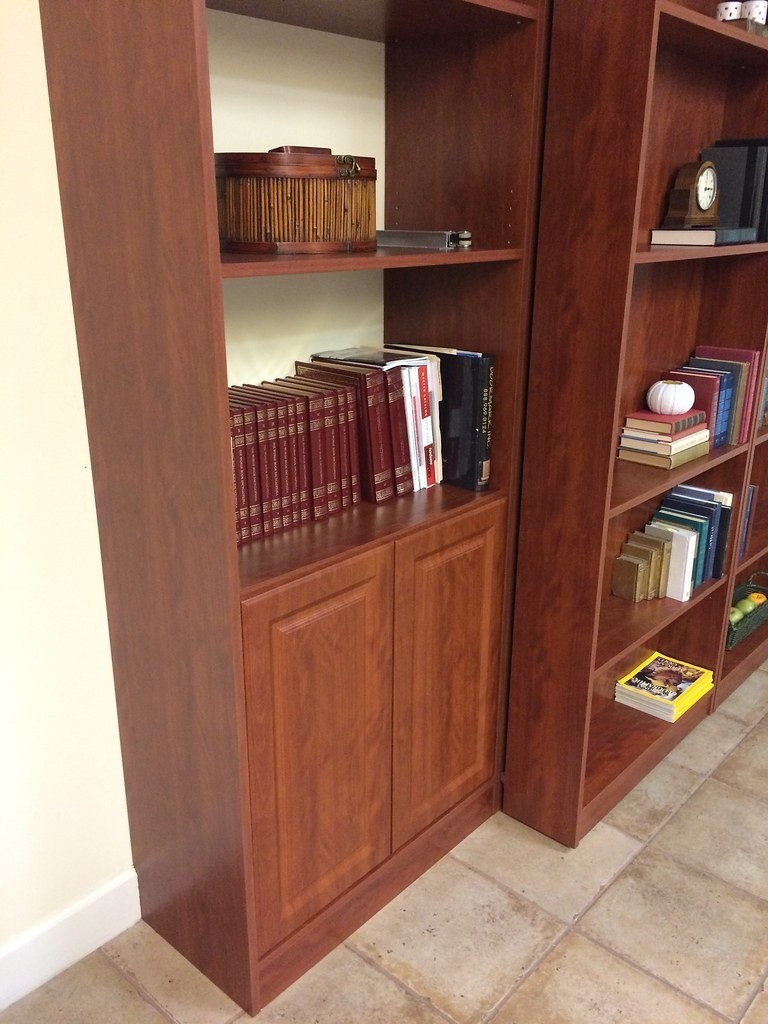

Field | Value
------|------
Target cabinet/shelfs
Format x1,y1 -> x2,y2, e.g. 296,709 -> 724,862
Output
497,0 -> 767,846
42,0 -> 550,1019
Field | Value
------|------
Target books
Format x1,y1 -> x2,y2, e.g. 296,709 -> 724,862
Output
739,485 -> 759,561
613,484 -> 733,603
618,429 -> 709,454
724,18 -> 768,39
615,651 -> 714,723
616,441 -> 714,470
624,409 -> 706,434
649,138 -> 768,246
663,344 -> 759,450
620,422 -> 707,442
229,343 -> 494,547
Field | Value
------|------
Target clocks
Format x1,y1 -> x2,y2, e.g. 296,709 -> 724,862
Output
660,161 -> 721,231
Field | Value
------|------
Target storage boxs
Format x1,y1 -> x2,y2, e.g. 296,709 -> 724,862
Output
214,146 -> 377,253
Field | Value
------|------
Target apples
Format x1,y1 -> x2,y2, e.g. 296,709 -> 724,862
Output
729,592 -> 768,624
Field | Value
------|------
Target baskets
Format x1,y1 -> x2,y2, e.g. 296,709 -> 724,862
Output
725,570 -> 768,649
